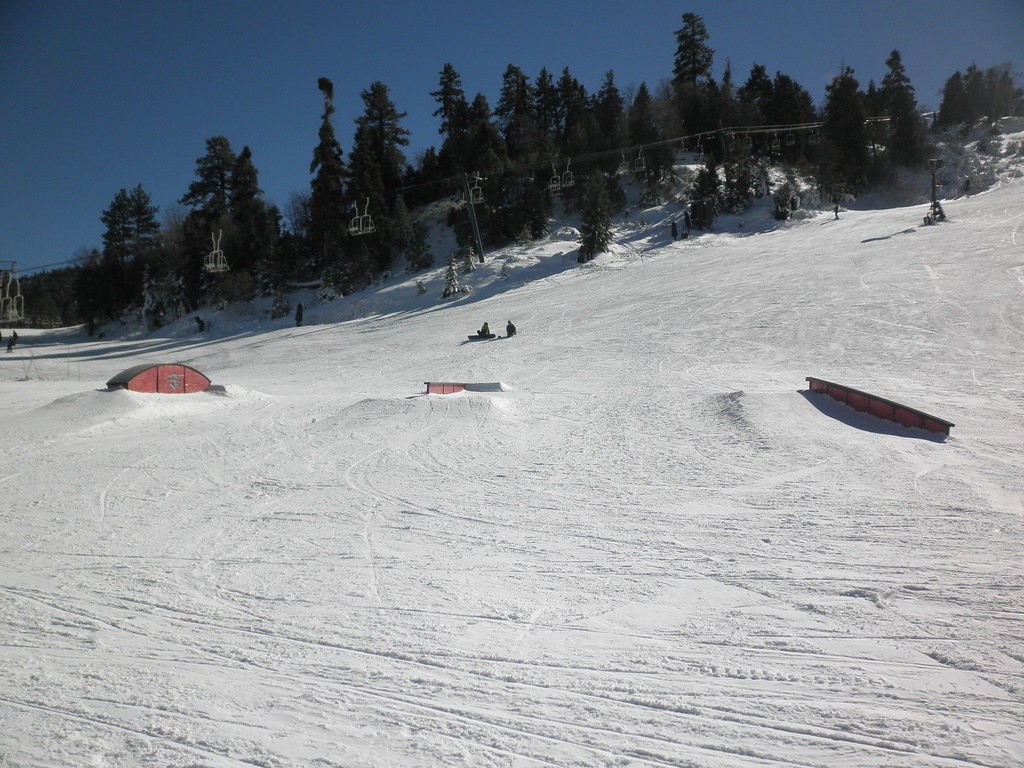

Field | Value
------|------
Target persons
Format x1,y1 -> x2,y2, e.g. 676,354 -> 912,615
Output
506,320 -> 516,338
194,316 -> 204,333
833,202 -> 839,220
671,222 -> 677,241
0,330 -> 18,353
295,303 -> 302,326
477,322 -> 490,336
685,210 -> 691,238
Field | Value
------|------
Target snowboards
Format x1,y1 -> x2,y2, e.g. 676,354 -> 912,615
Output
468,334 -> 496,339
